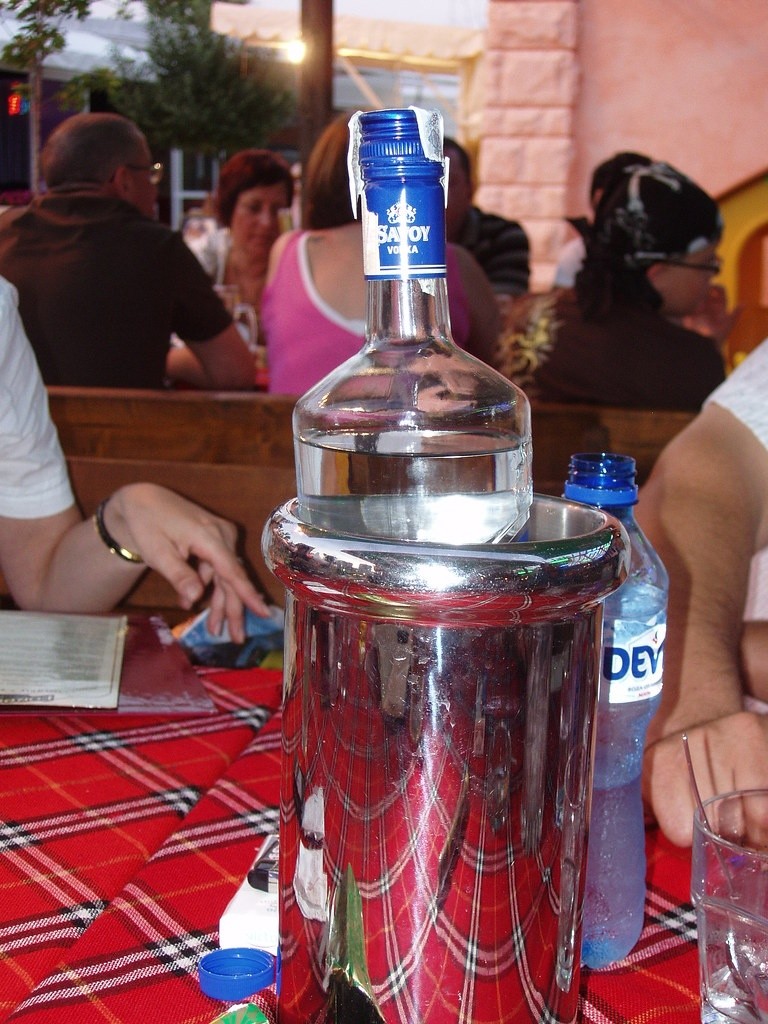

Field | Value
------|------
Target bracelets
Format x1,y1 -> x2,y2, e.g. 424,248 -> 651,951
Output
96,497 -> 145,564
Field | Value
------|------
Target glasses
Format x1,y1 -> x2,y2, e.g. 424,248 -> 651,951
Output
107,161 -> 162,185
640,255 -> 724,273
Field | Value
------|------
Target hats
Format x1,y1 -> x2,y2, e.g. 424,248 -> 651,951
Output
566,162 -> 723,265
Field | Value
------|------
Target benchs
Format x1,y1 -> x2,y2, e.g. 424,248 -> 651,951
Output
43,387 -> 700,611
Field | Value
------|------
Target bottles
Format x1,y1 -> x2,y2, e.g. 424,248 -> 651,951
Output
284,111 -> 540,545
546,452 -> 670,973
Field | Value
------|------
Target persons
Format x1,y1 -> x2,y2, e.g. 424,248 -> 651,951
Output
0,272 -> 274,644
0,114 -> 259,391
260,111 -> 531,396
633,336 -> 768,847
528,152 -> 744,414
167,148 -> 295,380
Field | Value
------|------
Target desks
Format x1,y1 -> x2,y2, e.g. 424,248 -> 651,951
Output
0,664 -> 708,1024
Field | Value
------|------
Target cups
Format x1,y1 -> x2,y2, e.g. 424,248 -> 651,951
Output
688,786 -> 768,1024
259,494 -> 631,1014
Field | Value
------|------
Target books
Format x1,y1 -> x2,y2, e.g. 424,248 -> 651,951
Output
0,610 -> 127,708
